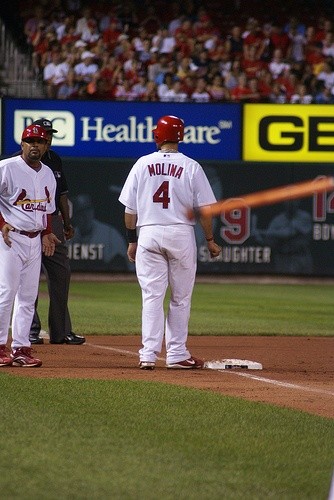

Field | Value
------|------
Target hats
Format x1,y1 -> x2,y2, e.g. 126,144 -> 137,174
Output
32,118 -> 58,133
21,124 -> 49,142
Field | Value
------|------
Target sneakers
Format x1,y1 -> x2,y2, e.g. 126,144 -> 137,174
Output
139,360 -> 157,370
166,356 -> 204,369
0,345 -> 14,367
11,347 -> 42,368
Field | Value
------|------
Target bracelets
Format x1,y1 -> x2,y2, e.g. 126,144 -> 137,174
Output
205,236 -> 215,242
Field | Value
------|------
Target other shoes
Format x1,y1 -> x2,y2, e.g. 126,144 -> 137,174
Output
49,332 -> 86,345
29,334 -> 44,344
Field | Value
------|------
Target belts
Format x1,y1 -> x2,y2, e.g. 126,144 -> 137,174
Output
11,228 -> 41,239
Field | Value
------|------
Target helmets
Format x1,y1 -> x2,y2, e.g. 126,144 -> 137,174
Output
152,115 -> 184,148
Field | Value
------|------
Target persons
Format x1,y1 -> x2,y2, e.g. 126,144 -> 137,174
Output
1,0 -> 334,104
12,119 -> 86,345
117,114 -> 221,368
0,124 -> 57,367
